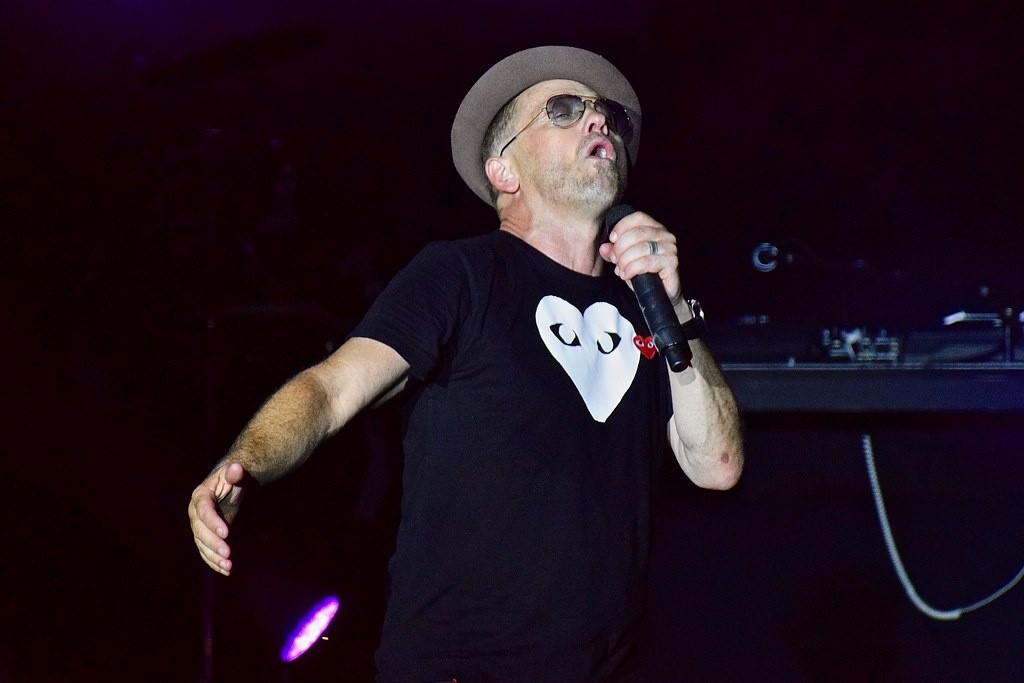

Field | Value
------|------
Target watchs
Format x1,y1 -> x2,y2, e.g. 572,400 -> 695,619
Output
680,299 -> 709,340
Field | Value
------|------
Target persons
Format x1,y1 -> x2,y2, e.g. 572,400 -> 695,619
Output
189,46 -> 741,683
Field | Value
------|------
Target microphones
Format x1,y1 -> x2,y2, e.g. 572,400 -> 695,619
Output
605,204 -> 692,374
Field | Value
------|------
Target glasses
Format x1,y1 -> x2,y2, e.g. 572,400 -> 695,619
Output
499,95 -> 635,158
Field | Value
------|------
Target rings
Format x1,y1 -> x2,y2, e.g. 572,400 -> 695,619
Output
648,241 -> 658,255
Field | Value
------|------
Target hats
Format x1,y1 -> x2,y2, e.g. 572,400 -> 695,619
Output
451,45 -> 642,207
